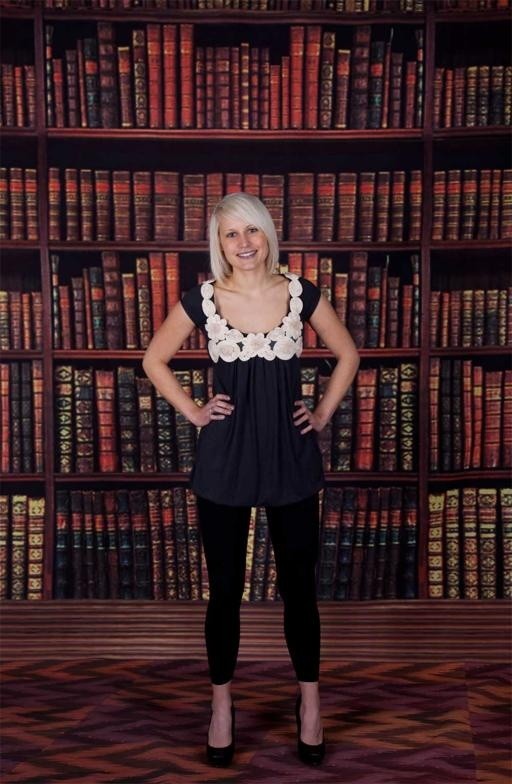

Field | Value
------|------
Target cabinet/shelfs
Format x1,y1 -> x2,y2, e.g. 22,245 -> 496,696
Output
1,0 -> 512,621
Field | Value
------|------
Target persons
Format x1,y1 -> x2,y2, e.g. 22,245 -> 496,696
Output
142,192 -> 361,768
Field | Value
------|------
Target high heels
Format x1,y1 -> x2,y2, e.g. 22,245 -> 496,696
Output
294,699 -> 327,766
206,700 -> 237,768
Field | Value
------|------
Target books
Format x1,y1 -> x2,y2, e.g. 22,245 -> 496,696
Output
1,251 -> 208,353
243,506 -> 281,601
0,358 -> 213,475
1,1 -> 512,128
0,166 -> 512,240
303,359 -> 511,474
0,484 -> 212,603
281,248 -> 512,353
317,486 -> 512,601
